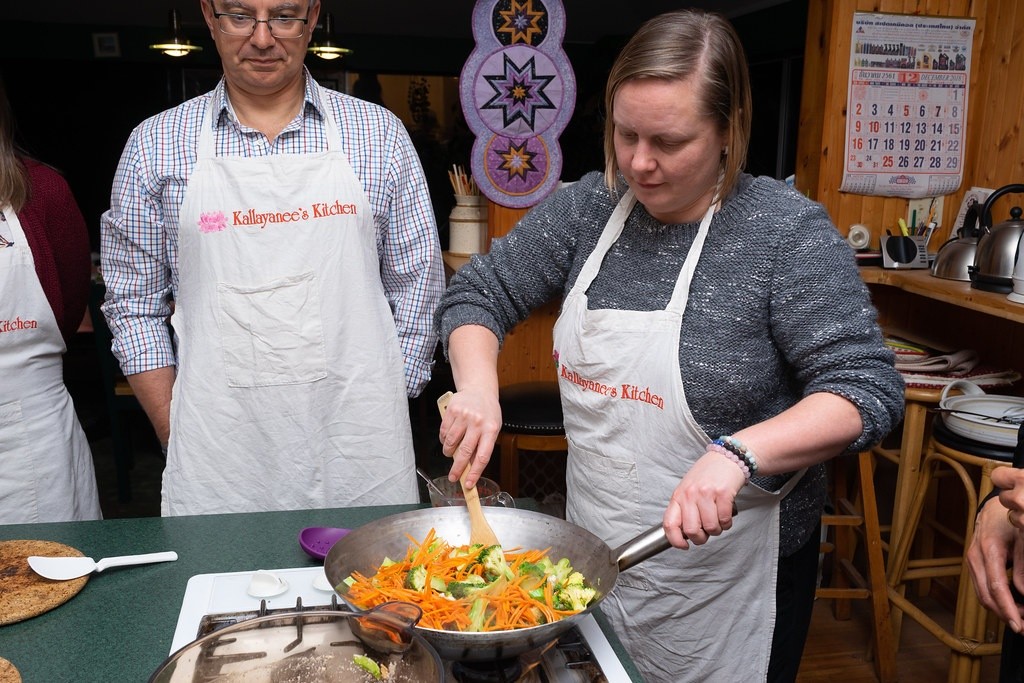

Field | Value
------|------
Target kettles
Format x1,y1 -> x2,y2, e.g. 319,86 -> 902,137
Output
929,204 -> 994,282
967,182 -> 1024,293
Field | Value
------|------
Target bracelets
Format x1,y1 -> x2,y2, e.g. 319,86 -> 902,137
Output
706,443 -> 750,486
711,438 -> 754,478
719,435 -> 758,475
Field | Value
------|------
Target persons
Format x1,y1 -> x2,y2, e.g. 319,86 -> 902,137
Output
98,1 -> 446,518
432,9 -> 906,683
0,108 -> 103,525
965,419 -> 1024,683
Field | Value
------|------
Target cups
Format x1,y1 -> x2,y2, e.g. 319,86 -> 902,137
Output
427,474 -> 515,507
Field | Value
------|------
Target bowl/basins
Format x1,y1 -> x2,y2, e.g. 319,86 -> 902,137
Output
148,595 -> 446,683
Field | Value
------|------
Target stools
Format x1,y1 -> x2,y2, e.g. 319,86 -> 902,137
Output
877,423 -> 1024,683
810,317 -> 1021,666
489,382 -> 572,500
809,427 -> 902,683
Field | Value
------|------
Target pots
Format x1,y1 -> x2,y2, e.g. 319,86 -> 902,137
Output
322,494 -> 741,669
939,377 -> 1024,448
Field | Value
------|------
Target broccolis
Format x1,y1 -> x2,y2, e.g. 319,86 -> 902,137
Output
404,542 -> 601,625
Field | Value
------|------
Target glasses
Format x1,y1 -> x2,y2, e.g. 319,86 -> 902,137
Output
211,0 -> 310,39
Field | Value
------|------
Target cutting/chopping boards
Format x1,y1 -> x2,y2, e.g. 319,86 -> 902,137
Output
1,538 -> 92,627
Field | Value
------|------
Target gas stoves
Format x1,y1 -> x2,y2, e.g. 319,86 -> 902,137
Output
166,562 -> 631,683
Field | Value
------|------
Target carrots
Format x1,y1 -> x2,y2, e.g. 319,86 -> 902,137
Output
339,527 -> 584,633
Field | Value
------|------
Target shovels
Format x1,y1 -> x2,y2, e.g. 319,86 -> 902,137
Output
27,552 -> 179,580
436,391 -> 500,552
934,406 -> 1024,425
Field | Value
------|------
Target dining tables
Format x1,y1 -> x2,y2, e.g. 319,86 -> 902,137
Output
0,489 -> 646,683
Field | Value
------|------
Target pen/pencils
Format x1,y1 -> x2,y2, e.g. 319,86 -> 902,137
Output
898,206 -> 937,246
